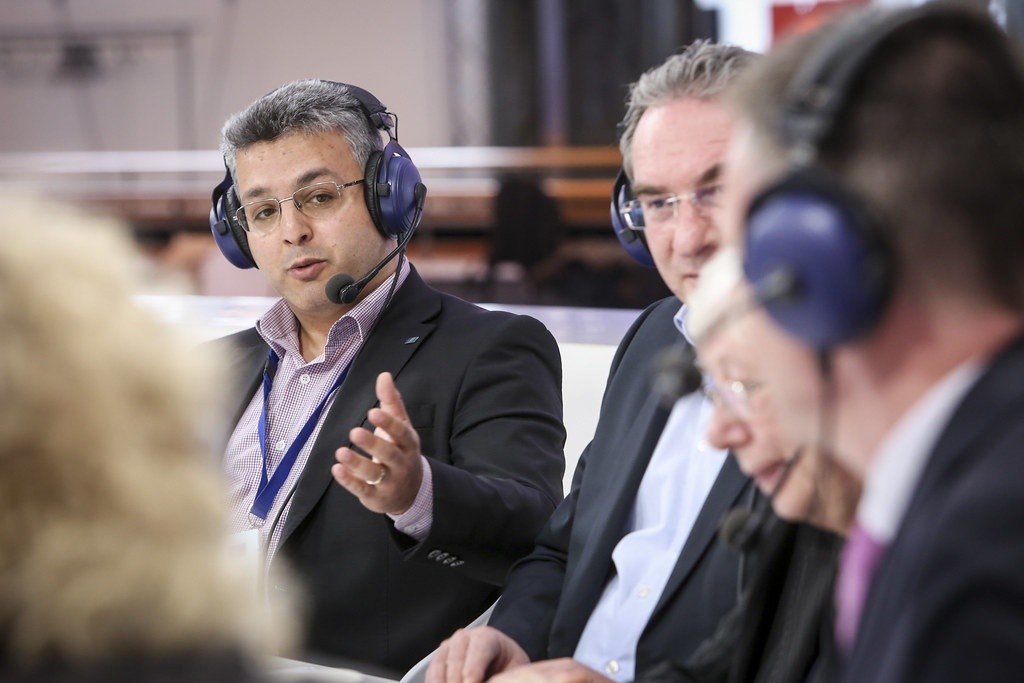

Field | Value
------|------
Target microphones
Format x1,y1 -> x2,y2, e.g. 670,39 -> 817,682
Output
325,208 -> 423,304
721,450 -> 802,552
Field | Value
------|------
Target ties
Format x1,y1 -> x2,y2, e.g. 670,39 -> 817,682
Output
831,518 -> 888,666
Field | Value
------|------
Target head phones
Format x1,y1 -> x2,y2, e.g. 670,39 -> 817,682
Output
739,0 -> 1007,355
209,83 -> 427,270
607,170 -> 659,269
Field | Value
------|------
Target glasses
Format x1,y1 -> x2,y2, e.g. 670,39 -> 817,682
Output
615,182 -> 735,232
232,176 -> 365,235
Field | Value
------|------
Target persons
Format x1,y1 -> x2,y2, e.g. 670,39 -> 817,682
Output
713,8 -> 1023,683
185,80 -> 568,683
684,247 -> 864,683
425,40 -> 782,683
0,199 -> 310,683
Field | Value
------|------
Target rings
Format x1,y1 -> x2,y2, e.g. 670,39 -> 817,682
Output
364,463 -> 386,485
